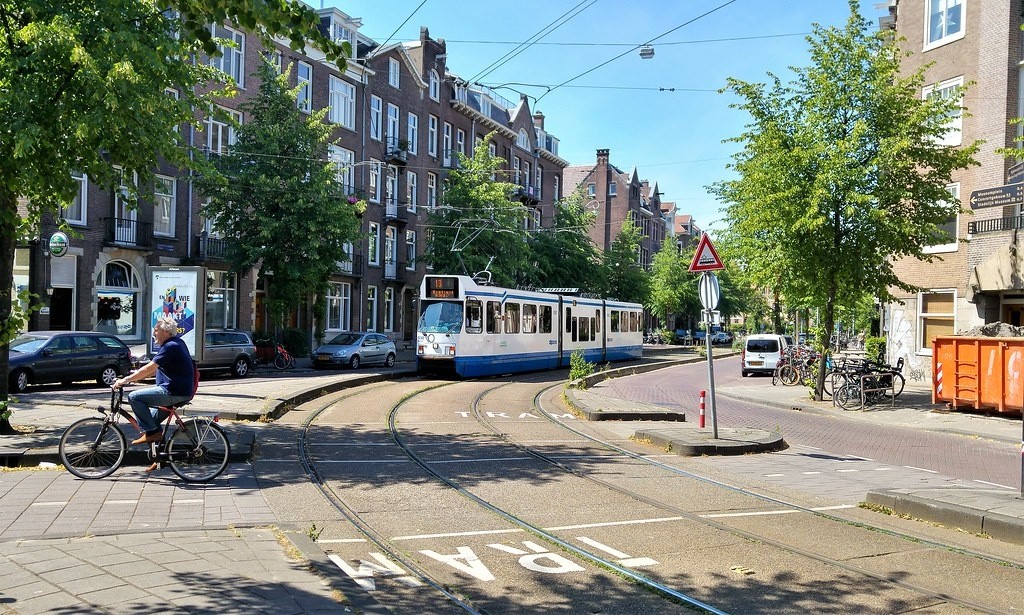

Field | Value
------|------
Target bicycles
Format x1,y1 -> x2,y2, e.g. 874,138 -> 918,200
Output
269,340 -> 297,370
57,377 -> 232,484
772,343 -> 907,412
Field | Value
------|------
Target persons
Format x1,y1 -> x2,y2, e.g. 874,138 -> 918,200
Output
111,317 -> 195,472
455,312 -> 469,327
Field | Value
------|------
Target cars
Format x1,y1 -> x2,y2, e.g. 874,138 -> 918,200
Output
310,332 -> 397,369
672,328 -> 733,345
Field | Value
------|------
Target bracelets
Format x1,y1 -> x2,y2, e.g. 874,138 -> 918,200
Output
124,376 -> 130,383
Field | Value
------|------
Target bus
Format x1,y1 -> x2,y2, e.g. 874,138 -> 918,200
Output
414,218 -> 645,380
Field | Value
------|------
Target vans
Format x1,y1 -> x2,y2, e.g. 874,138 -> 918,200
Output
741,334 -> 787,378
795,331 -> 816,344
779,335 -> 796,346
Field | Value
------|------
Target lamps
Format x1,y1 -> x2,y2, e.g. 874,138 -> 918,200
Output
639,46 -> 655,59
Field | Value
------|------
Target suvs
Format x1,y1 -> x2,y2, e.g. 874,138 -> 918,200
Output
128,327 -> 257,379
8,331 -> 134,392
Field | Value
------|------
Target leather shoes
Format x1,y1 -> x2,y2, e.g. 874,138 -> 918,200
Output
146,462 -> 157,472
132,431 -> 162,444
